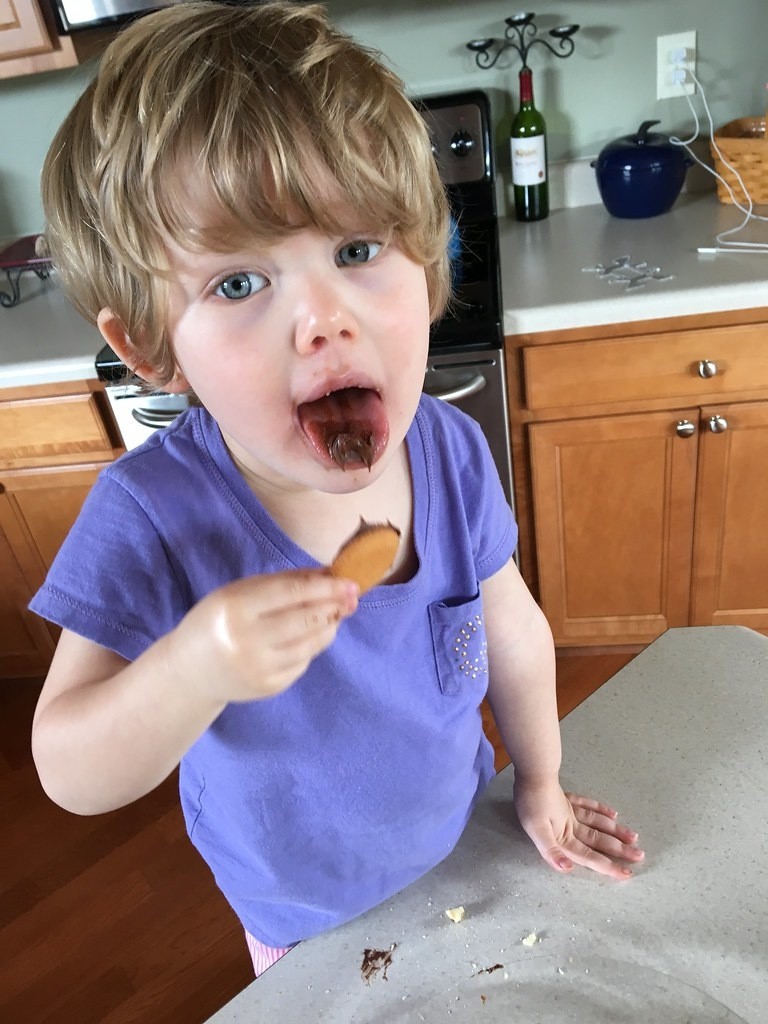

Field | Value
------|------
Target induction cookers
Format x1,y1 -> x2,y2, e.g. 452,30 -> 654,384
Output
93,86 -> 506,377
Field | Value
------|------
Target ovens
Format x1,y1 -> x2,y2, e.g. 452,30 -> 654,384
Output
102,348 -> 520,580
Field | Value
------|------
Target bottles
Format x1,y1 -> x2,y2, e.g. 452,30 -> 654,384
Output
508,67 -> 551,221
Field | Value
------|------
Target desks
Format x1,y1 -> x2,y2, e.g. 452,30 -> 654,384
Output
203,624 -> 768,1024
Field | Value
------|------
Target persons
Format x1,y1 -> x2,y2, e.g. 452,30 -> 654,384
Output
28,0 -> 644,977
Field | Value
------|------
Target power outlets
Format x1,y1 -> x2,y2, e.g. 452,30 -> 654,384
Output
656,29 -> 696,101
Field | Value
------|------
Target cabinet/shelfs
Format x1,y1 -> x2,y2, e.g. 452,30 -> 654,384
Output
0,381 -> 128,680
0,0 -> 119,79
503,305 -> 768,658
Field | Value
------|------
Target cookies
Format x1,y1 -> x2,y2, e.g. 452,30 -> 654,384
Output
329,521 -> 400,596
